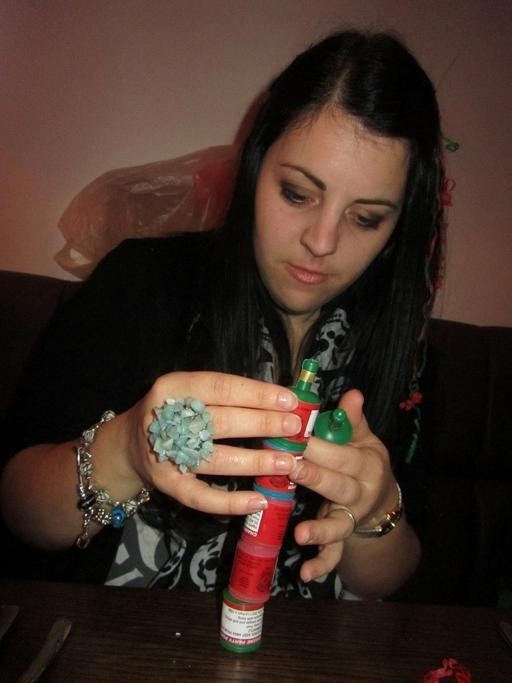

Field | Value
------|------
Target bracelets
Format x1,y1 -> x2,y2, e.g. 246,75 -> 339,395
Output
351,479 -> 406,537
72,409 -> 153,530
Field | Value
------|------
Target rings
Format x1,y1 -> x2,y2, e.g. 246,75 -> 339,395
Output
144,395 -> 219,476
323,508 -> 358,534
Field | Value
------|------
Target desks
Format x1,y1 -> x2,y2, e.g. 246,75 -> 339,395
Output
6,567 -> 511,682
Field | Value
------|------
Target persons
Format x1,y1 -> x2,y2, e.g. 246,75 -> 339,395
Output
1,27 -> 447,601
